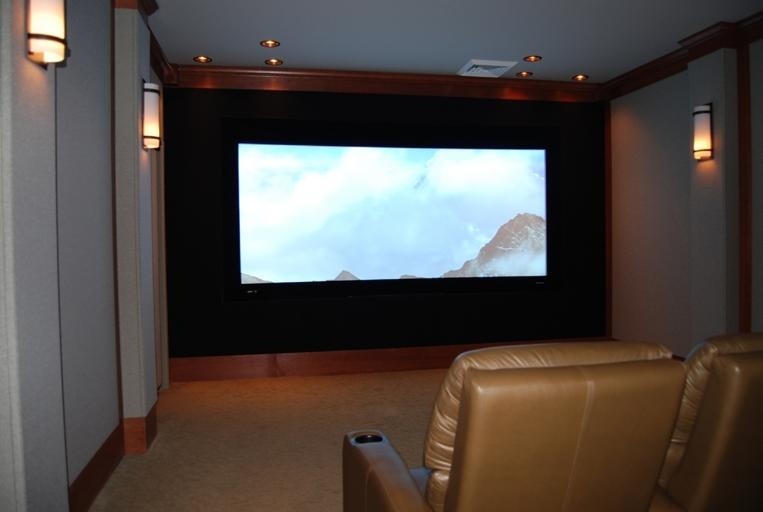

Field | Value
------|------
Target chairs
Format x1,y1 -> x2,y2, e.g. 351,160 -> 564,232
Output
655,335 -> 763,510
340,338 -> 689,512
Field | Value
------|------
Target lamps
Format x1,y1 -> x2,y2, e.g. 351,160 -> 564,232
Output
143,82 -> 161,150
692,105 -> 712,160
27,0 -> 65,64
